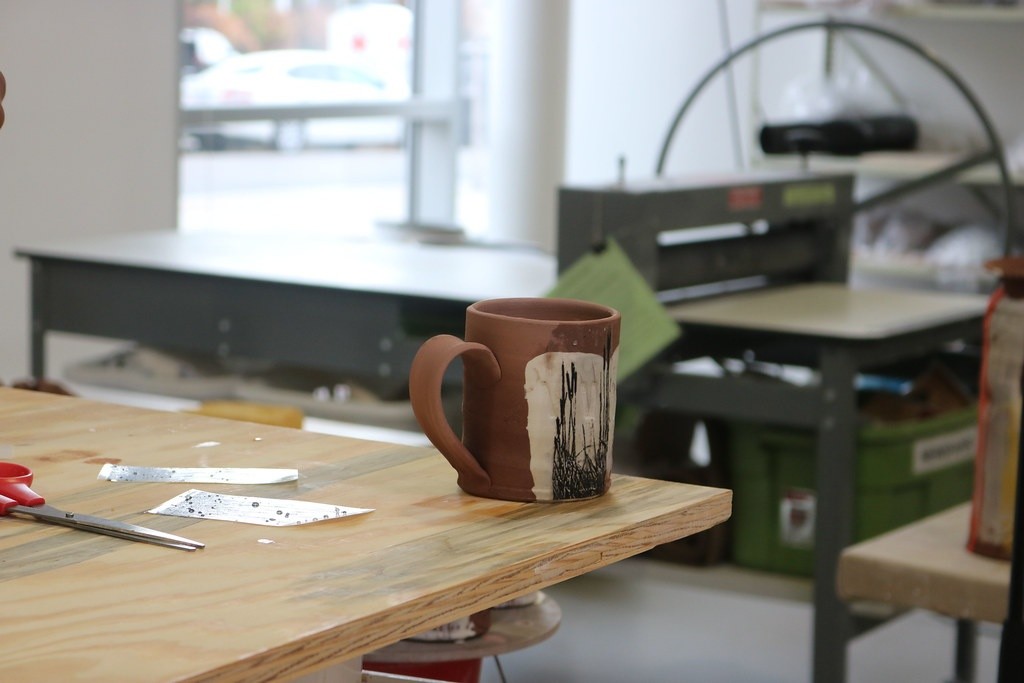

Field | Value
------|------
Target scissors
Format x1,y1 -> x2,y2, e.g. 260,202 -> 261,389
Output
0,459 -> 207,554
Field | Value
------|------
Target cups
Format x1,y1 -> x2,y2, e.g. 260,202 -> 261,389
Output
410,300 -> 621,503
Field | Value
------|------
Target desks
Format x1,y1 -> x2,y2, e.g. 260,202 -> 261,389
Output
15,239 -> 563,388
0,385 -> 733,683
620,282 -> 992,682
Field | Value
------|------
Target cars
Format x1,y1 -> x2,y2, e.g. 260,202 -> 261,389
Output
179,27 -> 242,76
179,50 -> 411,149
